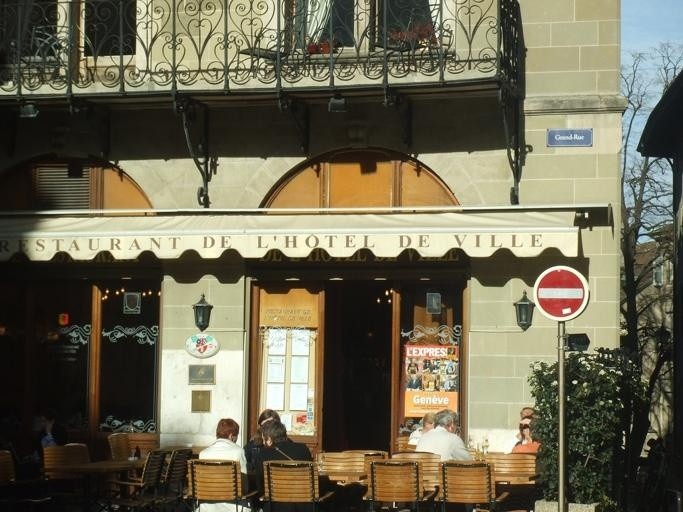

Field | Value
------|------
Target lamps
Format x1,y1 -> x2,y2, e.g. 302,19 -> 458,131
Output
193,294 -> 213,332
513,289 -> 535,330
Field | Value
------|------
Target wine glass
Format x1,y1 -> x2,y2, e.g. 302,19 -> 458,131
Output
314,449 -> 325,472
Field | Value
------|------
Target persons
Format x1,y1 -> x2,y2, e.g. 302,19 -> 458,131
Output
39,408 -> 58,447
195,418 -> 264,512
511,407 -> 541,454
243,409 -> 293,463
408,411 -> 435,446
414,408 -> 473,462
253,418 -> 313,496
407,359 -> 422,388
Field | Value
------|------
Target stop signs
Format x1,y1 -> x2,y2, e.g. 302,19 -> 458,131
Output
533,265 -> 590,322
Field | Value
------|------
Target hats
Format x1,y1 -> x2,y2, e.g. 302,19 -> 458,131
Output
520,407 -> 534,419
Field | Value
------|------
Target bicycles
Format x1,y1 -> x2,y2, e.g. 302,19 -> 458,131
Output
622,434 -> 680,512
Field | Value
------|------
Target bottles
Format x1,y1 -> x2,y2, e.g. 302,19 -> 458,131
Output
472,437 -> 490,463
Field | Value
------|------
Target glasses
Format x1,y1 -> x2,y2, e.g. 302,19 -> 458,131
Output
519,423 -> 529,429
